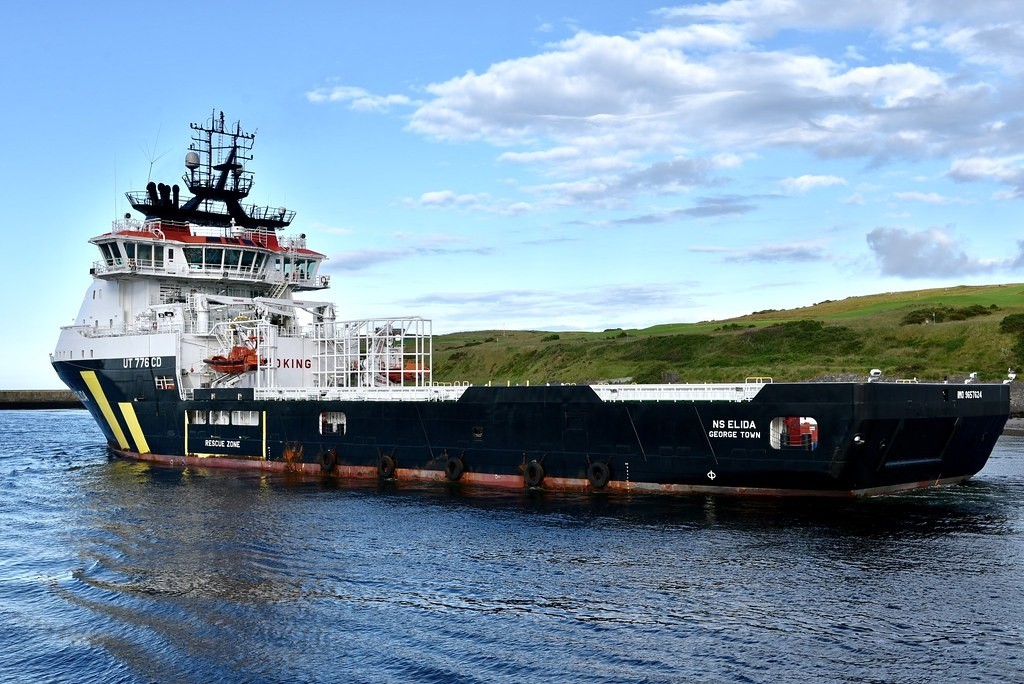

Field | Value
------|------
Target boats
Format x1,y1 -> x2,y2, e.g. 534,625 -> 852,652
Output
49,108 -> 1017,499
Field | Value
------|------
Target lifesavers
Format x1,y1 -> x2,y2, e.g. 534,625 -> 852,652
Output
587,461 -> 610,486
379,456 -> 395,477
321,449 -> 335,470
445,458 -> 463,480
525,460 -> 544,486
